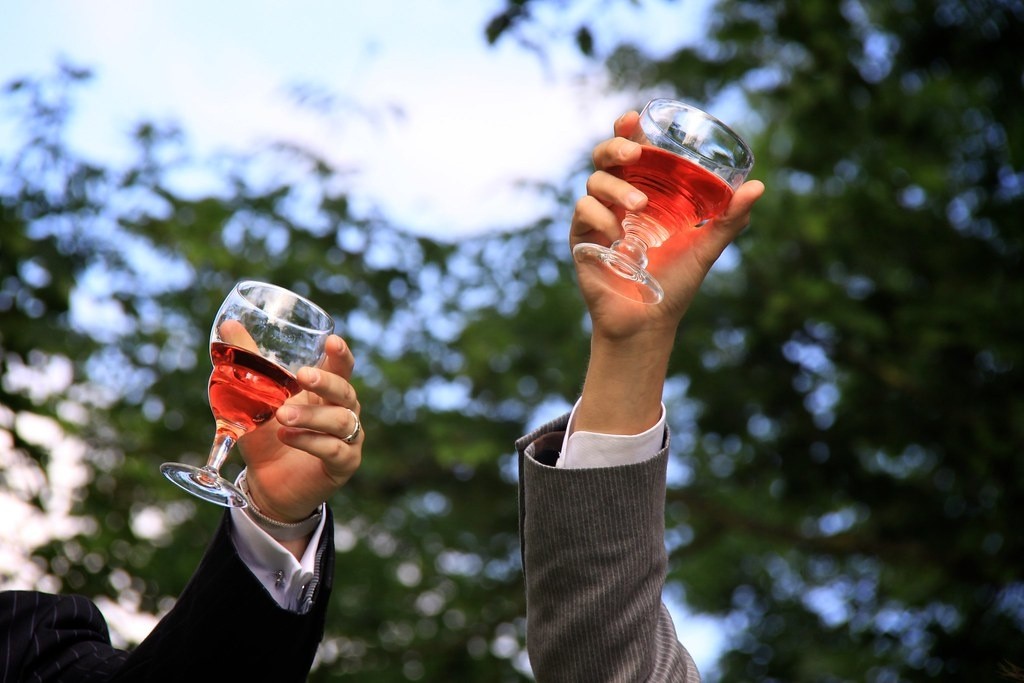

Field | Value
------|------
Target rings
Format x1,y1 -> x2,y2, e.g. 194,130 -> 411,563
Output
339,408 -> 361,444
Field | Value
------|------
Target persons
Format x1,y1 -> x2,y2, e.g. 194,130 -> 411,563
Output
519,109 -> 765,683
0,318 -> 364,683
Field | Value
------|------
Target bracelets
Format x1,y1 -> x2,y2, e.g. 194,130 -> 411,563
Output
238,471 -> 321,540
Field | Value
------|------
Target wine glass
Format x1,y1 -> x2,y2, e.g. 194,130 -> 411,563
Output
159,281 -> 336,508
571,99 -> 755,304
